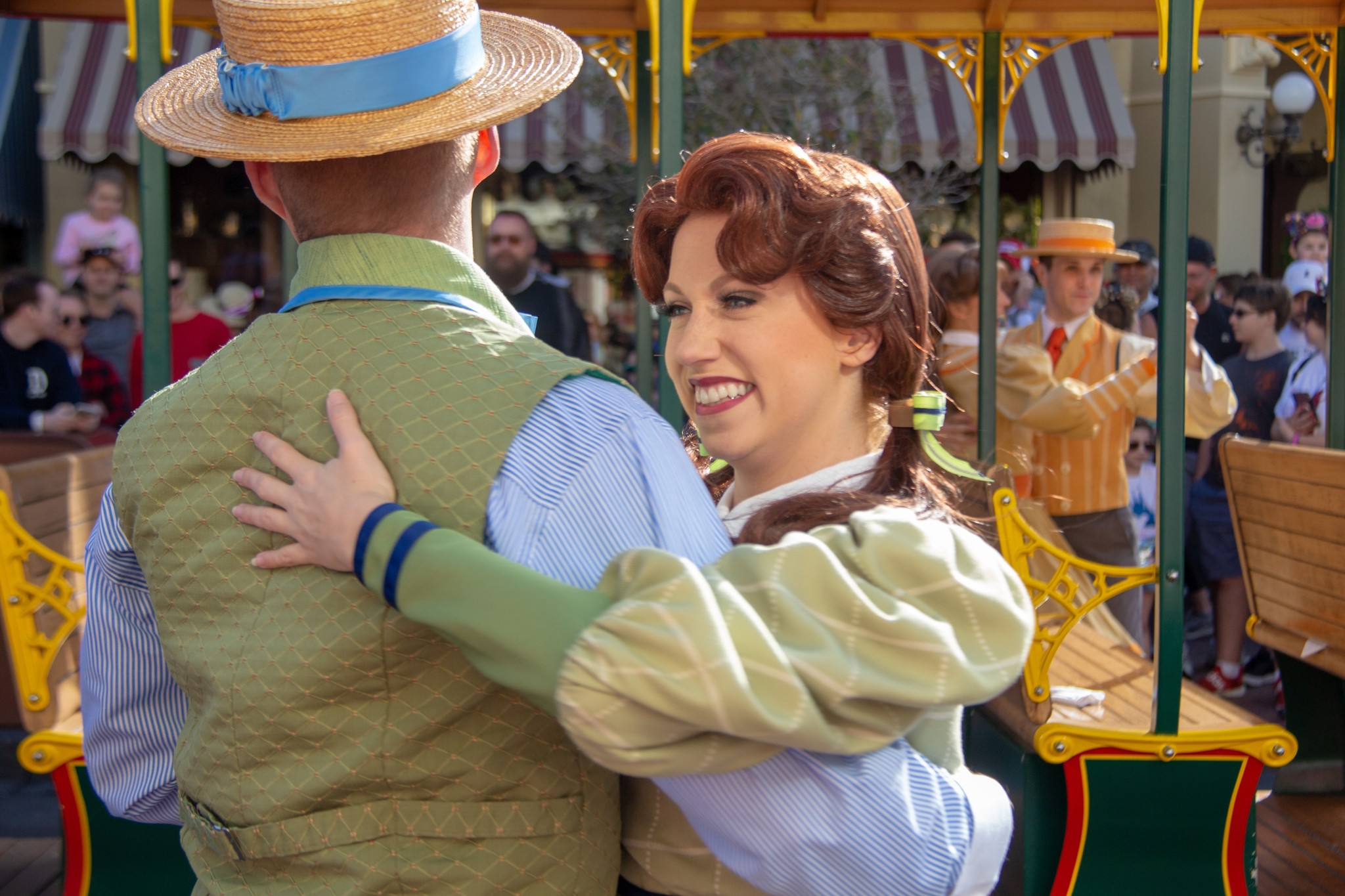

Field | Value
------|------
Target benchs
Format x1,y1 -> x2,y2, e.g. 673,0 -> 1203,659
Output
912,451 -> 1300,896
0,439 -> 198,896
1217,431 -> 1345,804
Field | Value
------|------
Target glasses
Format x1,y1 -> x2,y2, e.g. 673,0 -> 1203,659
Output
485,232 -> 525,246
56,311 -> 94,328
1127,440 -> 1154,452
168,276 -> 182,288
1232,308 -> 1252,318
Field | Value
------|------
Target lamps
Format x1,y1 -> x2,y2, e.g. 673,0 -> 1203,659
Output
1235,73 -> 1317,172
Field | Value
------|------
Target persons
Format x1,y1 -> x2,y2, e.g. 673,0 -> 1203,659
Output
78,0 -> 1014,895
481,213 -> 591,359
0,166 -> 257,439
935,217 -> 1329,707
228,131 -> 1039,896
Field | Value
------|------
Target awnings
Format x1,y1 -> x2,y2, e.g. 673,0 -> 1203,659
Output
0,15 -> 1137,231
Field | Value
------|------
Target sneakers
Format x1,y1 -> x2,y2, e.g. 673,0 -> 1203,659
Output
1195,662 -> 1247,698
1242,650 -> 1278,687
1185,613 -> 1214,640
1274,676 -> 1287,717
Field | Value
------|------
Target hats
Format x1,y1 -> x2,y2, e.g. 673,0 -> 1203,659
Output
1186,234 -> 1216,266
1114,238 -> 1157,266
998,238 -> 1032,275
1282,258 -> 1327,300
1009,219 -> 1141,264
135,6 -> 585,162
